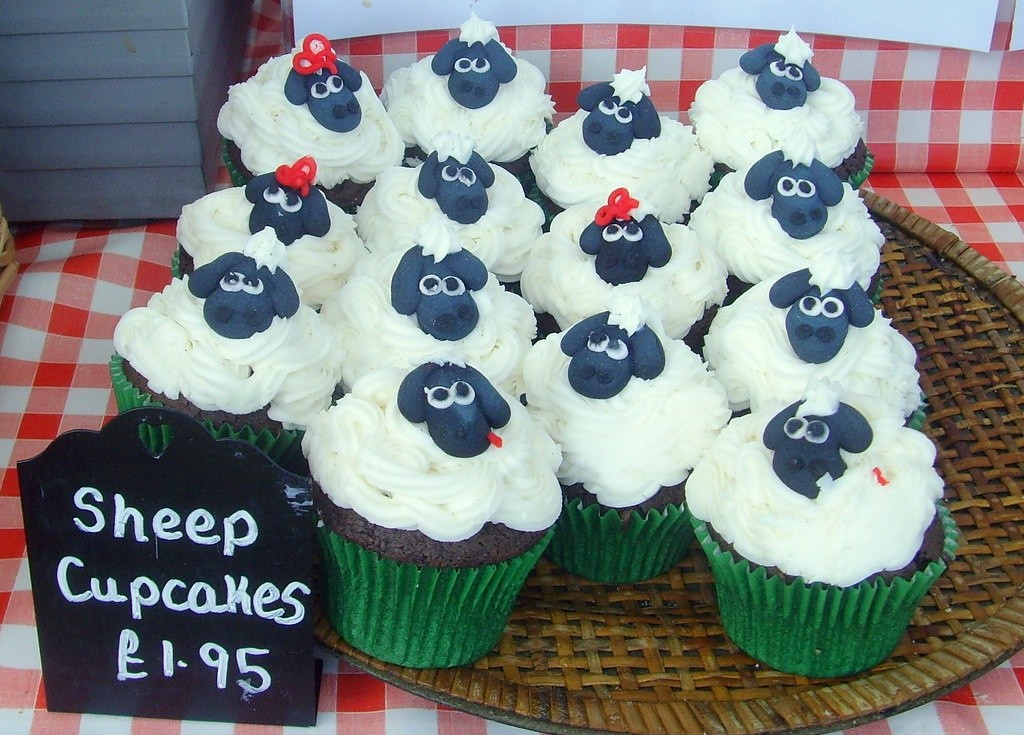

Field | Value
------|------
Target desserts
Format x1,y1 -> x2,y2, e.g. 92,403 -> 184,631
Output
112,7 -> 962,673
